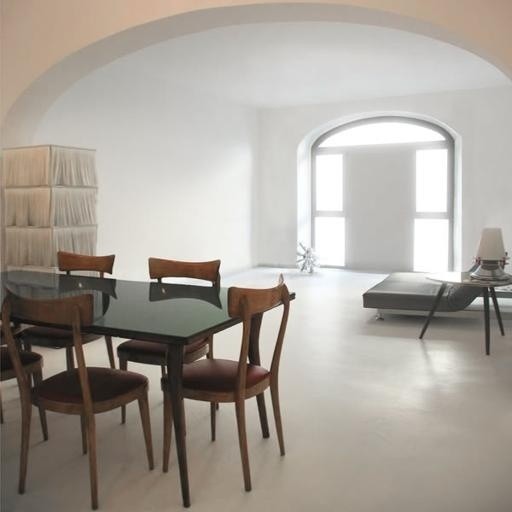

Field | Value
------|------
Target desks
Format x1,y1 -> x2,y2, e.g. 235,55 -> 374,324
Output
419,272 -> 511,355
1,263 -> 301,509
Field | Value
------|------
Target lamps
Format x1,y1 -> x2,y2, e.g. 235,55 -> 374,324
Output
469,227 -> 510,281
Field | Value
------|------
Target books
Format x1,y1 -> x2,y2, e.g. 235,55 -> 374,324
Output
1,146 -> 104,322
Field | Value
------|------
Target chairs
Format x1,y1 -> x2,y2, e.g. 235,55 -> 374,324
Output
114,256 -> 223,442
19,250 -> 118,429
160,271 -> 293,492
1,348 -> 49,443
362,251 -> 511,322
1,283 -> 157,512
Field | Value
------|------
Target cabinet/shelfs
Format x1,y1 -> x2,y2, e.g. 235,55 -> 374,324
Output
1,144 -> 98,275
